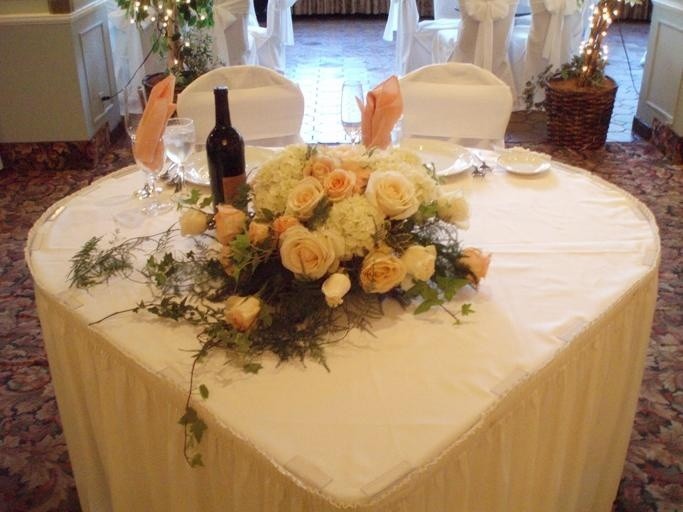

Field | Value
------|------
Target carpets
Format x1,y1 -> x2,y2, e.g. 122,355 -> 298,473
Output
0,118 -> 681,512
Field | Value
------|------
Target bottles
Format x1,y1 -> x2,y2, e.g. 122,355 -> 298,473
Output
206,85 -> 246,209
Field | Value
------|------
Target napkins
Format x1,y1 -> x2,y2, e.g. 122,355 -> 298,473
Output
362,77 -> 403,146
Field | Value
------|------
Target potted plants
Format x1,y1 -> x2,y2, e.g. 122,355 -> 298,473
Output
542,2 -> 625,148
117,1 -> 216,108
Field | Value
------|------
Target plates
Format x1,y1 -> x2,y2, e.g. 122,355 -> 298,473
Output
389,138 -> 478,176
499,152 -> 550,175
180,145 -> 280,187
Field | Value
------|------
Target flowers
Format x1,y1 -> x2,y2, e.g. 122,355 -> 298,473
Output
74,142 -> 491,471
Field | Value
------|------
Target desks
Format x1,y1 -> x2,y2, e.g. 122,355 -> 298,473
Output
404,18 -> 530,70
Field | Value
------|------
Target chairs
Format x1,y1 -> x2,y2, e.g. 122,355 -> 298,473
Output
385,2 -> 429,72
251,1 -> 297,69
515,1 -> 594,110
178,64 -> 303,142
434,2 -> 518,107
401,62 -> 515,149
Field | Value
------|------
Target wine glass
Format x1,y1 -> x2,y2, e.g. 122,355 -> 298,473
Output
164,117 -> 197,204
124,86 -> 175,217
340,81 -> 364,145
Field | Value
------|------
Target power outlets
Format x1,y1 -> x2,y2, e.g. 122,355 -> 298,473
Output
99,93 -> 106,112
